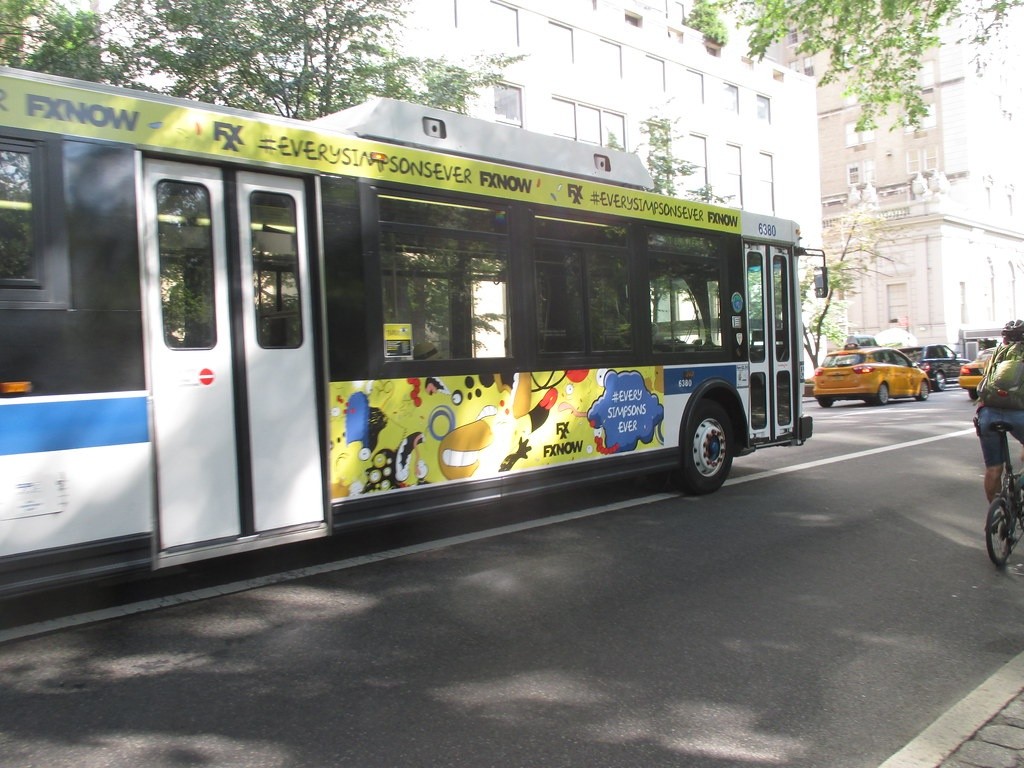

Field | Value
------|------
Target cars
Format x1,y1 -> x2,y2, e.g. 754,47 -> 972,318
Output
813,345 -> 931,409
899,345 -> 976,393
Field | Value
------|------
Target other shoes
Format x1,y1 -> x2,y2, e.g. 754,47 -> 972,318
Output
990,511 -> 1002,526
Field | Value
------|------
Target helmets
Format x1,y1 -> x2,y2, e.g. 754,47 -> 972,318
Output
1000,320 -> 1024,336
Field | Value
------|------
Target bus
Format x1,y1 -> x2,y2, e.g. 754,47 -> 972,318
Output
0,69 -> 828,597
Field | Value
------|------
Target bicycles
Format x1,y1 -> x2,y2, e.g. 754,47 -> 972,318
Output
985,422 -> 1023,565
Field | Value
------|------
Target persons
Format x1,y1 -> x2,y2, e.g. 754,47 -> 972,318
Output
545,266 -> 601,349
977,320 -> 1024,512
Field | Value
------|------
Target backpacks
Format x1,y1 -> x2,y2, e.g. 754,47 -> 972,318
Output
977,340 -> 1024,410
